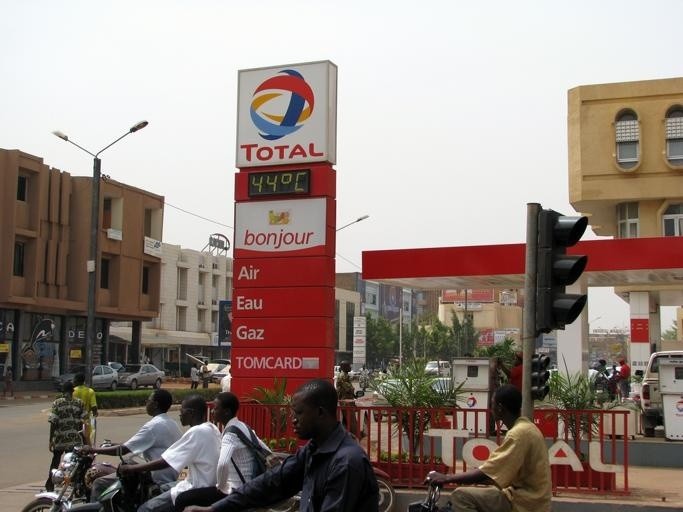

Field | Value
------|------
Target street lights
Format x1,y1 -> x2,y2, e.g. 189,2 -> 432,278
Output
52,119 -> 149,389
335,212 -> 369,232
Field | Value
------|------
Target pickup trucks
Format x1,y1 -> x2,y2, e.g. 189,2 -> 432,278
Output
637,347 -> 683,437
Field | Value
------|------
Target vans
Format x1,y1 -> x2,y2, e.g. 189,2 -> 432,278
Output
421,359 -> 450,378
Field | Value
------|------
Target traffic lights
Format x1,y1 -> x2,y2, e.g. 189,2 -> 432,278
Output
530,354 -> 549,400
535,202 -> 588,334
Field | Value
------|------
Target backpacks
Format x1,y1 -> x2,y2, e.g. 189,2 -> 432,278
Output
221,422 -> 281,484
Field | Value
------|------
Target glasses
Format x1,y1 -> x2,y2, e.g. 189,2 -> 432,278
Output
181,408 -> 194,414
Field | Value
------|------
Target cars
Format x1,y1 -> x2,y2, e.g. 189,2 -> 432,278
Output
372,377 -> 422,423
212,364 -> 230,383
426,374 -> 455,409
331,363 -> 360,382
51,361 -> 165,390
197,362 -> 226,381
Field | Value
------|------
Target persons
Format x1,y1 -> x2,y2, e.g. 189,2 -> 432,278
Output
220,367 -> 232,392
596,360 -> 609,389
422,385 -> 552,511
617,359 -> 631,403
1,366 -> 14,398
43,381 -> 90,492
190,362 -> 200,390
356,364 -> 370,391
71,374 -> 99,447
336,360 -> 360,439
498,350 -> 523,394
221,309 -> 234,332
203,361 -> 211,388
174,392 -> 276,511
78,390 -> 184,512
117,396 -> 223,512
183,378 -> 380,512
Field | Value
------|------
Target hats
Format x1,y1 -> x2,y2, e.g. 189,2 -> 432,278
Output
516,350 -> 523,360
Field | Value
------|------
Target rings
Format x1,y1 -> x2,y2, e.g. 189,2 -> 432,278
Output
426,476 -> 431,481
